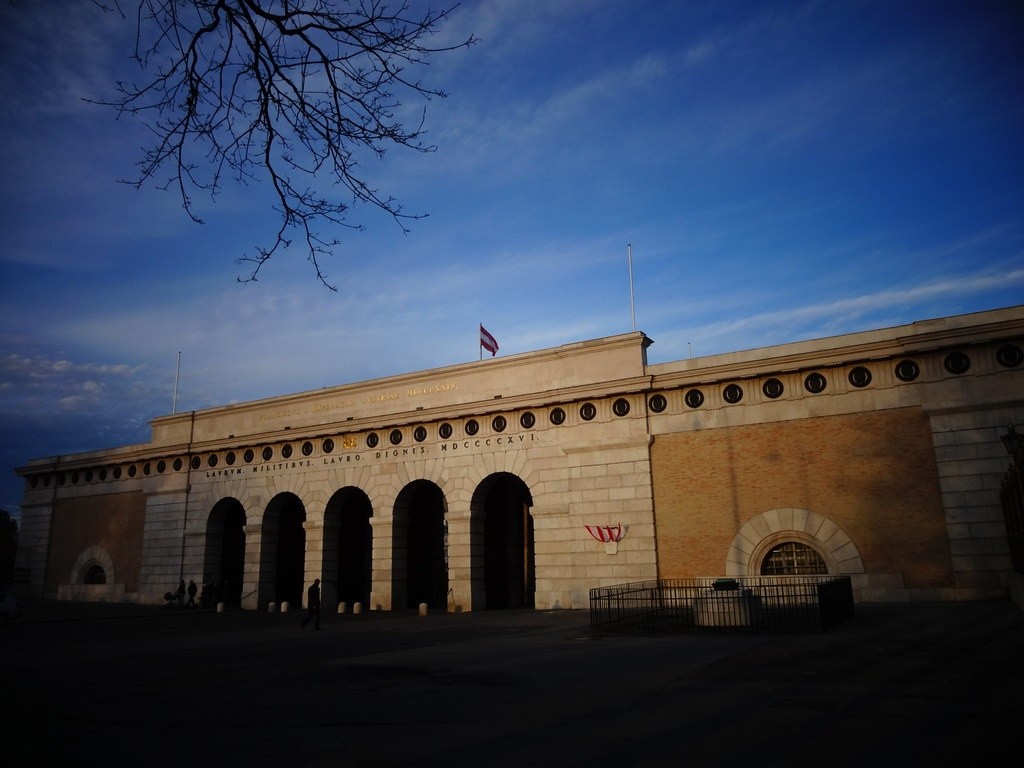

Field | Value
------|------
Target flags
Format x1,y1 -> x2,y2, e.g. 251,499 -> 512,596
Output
480,324 -> 499,358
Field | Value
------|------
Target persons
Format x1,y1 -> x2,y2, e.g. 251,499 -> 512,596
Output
199,580 -> 234,608
301,579 -> 323,631
175,580 -> 186,608
184,580 -> 197,610
